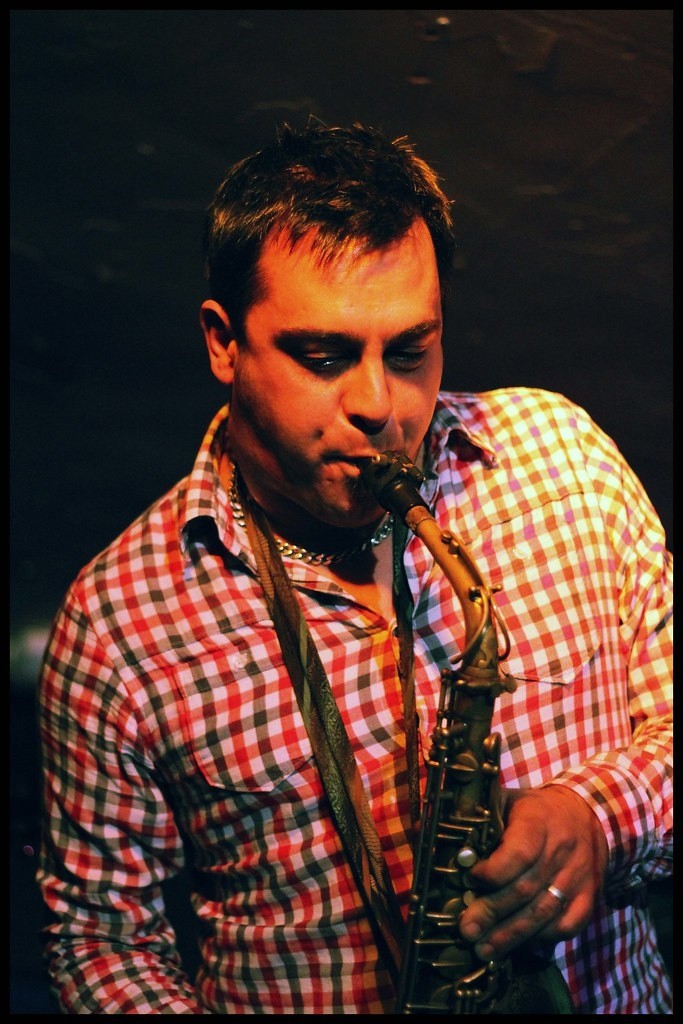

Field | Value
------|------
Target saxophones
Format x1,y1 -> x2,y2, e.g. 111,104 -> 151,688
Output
345,445 -> 536,1014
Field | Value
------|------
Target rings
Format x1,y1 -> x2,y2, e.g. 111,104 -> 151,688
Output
542,882 -> 568,913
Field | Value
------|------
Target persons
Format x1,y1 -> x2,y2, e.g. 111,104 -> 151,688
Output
38,109 -> 673,1014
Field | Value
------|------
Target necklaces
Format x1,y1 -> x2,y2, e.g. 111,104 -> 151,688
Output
229,443 -> 422,567
